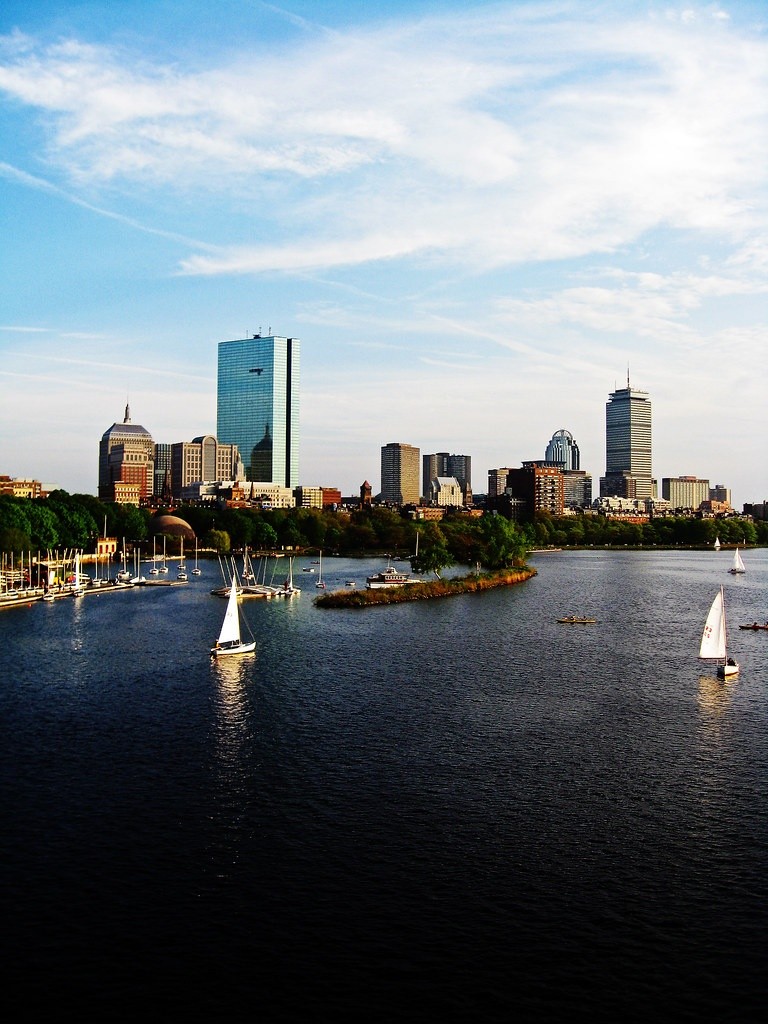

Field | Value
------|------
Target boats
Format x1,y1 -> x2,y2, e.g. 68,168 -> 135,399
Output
364,559 -> 427,590
737,621 -> 768,631
344,580 -> 357,587
556,614 -> 596,624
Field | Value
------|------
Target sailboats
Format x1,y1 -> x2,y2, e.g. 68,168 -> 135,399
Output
210,552 -> 302,597
190,537 -> 201,576
209,575 -> 257,656
314,550 -> 326,589
176,535 -> 187,581
158,536 -> 169,574
2,551 -> 89,599
90,537 -> 147,590
148,536 -> 159,575
40,553 -> 55,602
70,554 -> 84,598
713,537 -> 721,551
694,584 -> 740,681
727,548 -> 748,575
